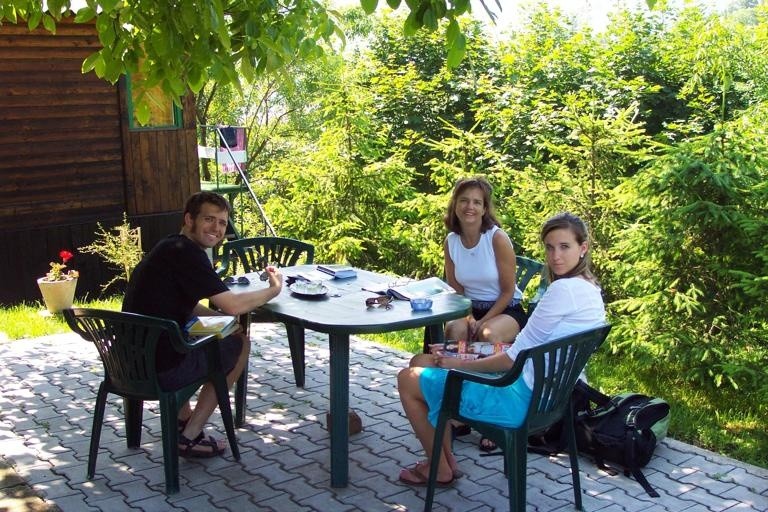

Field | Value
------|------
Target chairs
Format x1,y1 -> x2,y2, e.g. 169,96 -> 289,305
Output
61,309 -> 241,495
422,255 -> 549,357
424,325 -> 615,511
216,238 -> 316,387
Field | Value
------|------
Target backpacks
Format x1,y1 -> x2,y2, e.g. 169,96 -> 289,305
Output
576,393 -> 670,497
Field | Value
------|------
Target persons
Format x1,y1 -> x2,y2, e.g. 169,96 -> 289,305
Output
121,191 -> 282,456
401,212 -> 605,486
443,176 -> 527,450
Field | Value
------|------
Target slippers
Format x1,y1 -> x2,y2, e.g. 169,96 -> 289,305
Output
416,458 -> 465,480
399,467 -> 459,488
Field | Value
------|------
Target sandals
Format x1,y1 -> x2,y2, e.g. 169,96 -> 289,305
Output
478,435 -> 498,451
450,422 -> 472,436
178,430 -> 226,459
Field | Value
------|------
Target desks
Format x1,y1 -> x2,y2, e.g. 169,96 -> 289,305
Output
219,265 -> 471,491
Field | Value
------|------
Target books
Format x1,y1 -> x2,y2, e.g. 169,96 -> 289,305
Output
185,315 -> 240,340
428,340 -> 513,360
362,276 -> 457,302
298,264 -> 357,282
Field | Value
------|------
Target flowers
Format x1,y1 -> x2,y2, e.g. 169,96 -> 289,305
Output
47,251 -> 79,281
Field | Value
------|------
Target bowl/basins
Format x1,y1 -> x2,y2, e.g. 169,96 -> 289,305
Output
409,298 -> 433,311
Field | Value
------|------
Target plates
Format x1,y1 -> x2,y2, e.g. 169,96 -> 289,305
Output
289,282 -> 328,296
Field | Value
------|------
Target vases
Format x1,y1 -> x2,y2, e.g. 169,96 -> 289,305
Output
37,275 -> 77,314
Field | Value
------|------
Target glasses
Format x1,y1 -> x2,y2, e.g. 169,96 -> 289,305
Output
223,276 -> 251,285
245,246 -> 270,280
365,294 -> 395,309
